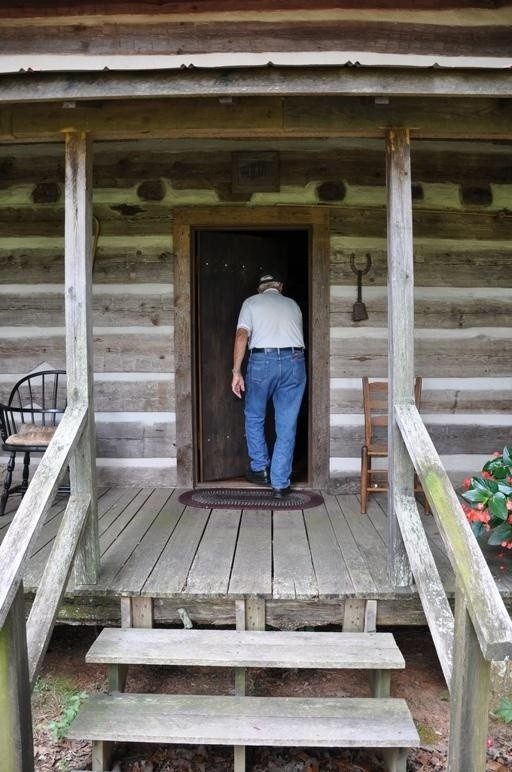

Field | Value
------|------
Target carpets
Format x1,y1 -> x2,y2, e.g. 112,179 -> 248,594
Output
176,487 -> 326,512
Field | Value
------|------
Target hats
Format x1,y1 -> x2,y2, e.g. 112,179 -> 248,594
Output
254,269 -> 283,286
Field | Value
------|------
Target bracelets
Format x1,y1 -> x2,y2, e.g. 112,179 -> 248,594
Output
231,368 -> 241,373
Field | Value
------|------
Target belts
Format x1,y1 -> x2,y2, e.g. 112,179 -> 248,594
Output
250,346 -> 305,354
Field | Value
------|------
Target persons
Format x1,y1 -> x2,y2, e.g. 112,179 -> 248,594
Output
230,273 -> 308,500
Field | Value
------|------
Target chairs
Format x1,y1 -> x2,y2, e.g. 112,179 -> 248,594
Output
357,371 -> 424,515
1,368 -> 71,517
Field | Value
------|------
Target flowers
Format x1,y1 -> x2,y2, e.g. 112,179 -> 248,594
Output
453,444 -> 512,554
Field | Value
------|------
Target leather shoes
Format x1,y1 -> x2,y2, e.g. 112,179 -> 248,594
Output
272,486 -> 294,499
247,469 -> 270,484
